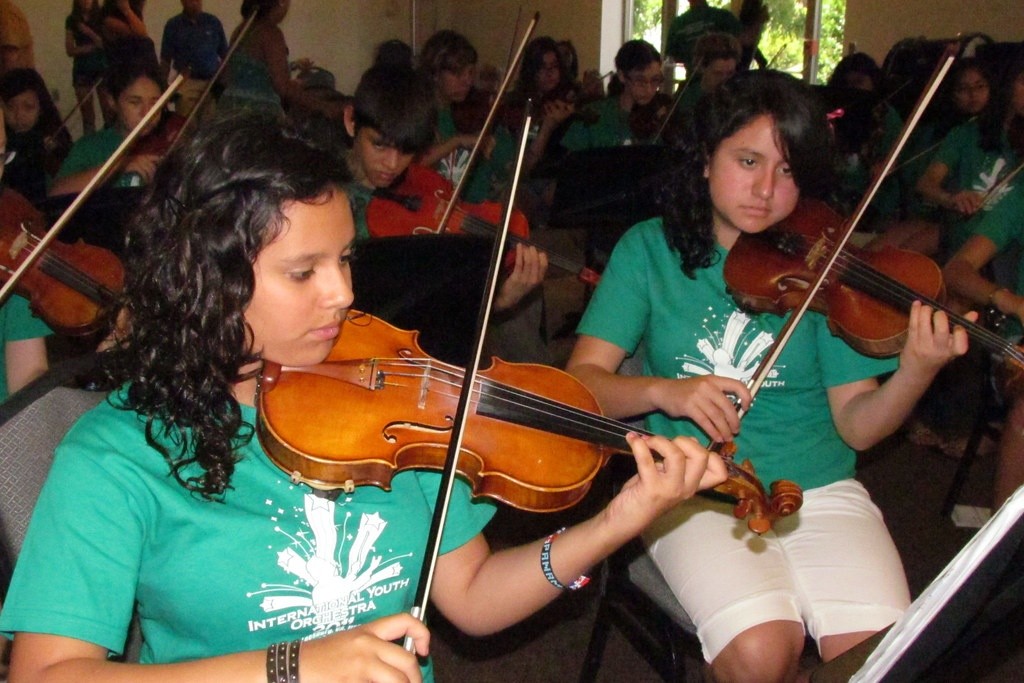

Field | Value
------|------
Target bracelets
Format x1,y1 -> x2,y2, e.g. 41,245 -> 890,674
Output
540,527 -> 595,592
266,639 -> 302,683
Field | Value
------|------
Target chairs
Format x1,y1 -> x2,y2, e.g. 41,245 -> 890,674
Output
572,465 -> 712,681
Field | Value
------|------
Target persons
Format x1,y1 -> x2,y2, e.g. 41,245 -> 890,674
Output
1,105 -> 729,683
0,0 -> 1024,515
564,68 -> 978,683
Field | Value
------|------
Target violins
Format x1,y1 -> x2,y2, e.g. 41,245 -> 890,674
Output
0,182 -> 134,340
723,183 -> 1024,382
361,159 -> 603,287
255,289 -> 803,542
0,38 -> 1023,219
